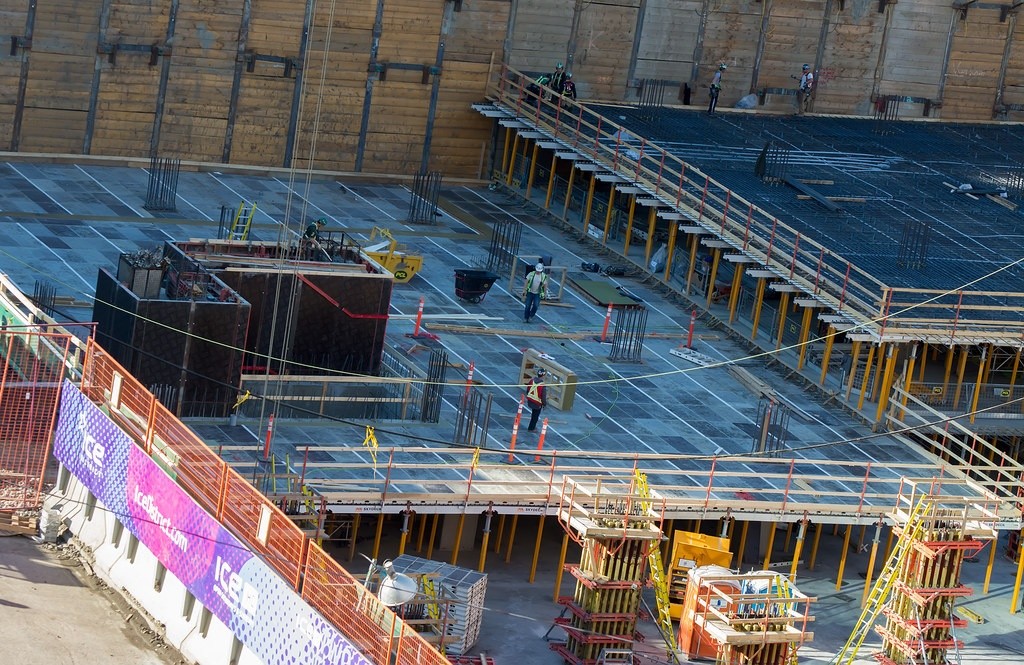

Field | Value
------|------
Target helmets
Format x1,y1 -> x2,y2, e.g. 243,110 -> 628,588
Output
535,263 -> 543,272
719,63 -> 726,69
320,218 -> 327,225
802,64 -> 810,69
537,368 -> 546,377
565,72 -> 572,78
556,62 -> 562,68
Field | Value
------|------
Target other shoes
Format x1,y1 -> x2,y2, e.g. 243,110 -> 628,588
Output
528,317 -> 532,322
524,318 -> 528,323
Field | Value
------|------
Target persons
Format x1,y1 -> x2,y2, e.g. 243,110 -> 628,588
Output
550,62 -> 566,113
526,367 -> 547,433
707,62 -> 726,117
791,64 -> 814,117
524,73 -> 553,107
557,72 -> 577,113
522,263 -> 547,323
303,218 -> 327,261
